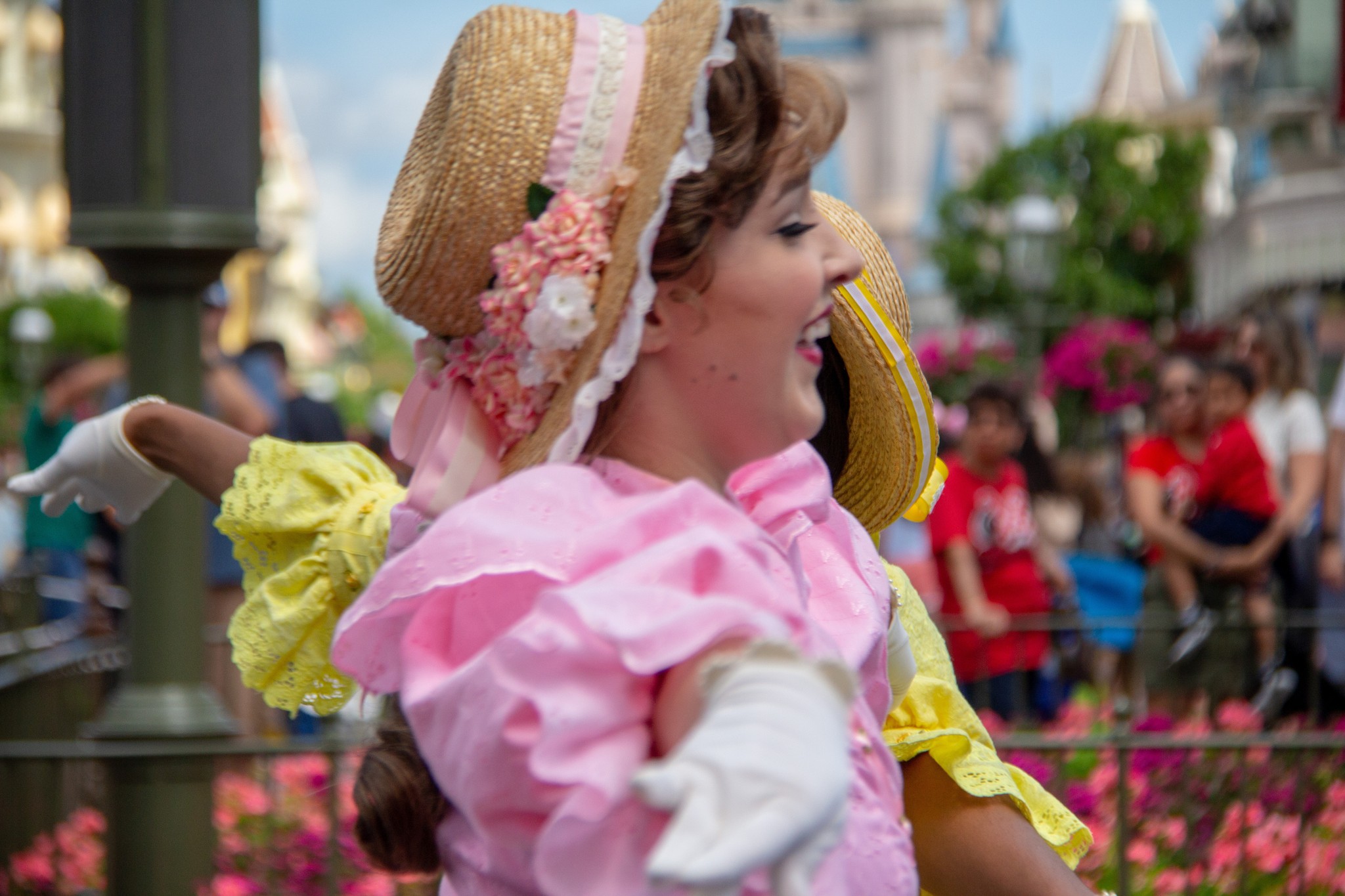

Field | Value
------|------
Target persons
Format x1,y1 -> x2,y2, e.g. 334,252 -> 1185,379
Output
338,6 -> 920,896
8,190 -> 1090,896
916,293 -> 1343,739
26,282 -> 413,748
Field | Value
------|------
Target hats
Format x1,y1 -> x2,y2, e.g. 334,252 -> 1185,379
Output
810,184 -> 940,536
372,0 -> 734,558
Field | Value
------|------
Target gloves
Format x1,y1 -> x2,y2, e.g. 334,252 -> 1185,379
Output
634,633 -> 863,895
7,394 -> 179,529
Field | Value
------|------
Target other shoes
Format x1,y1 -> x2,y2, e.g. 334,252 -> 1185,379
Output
1254,661 -> 1298,723
1171,605 -> 1216,664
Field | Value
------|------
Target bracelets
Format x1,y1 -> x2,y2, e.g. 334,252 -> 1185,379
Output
200,351 -> 229,371
1209,543 -> 1226,576
